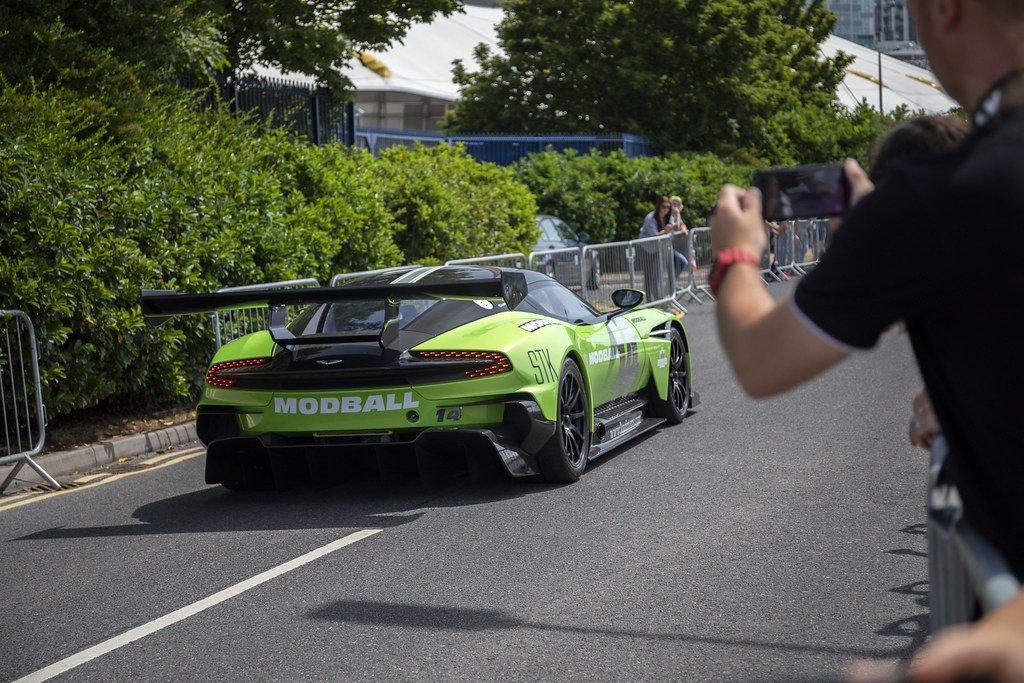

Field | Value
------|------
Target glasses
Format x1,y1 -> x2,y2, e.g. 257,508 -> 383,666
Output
661,204 -> 671,210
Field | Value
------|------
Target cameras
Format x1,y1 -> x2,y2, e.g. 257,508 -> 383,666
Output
751,165 -> 849,221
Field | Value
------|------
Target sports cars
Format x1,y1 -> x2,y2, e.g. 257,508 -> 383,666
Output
140,266 -> 693,493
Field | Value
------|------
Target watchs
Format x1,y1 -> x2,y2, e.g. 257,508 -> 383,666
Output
707,247 -> 762,299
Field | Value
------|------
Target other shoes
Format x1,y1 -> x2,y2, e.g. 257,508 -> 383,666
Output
764,273 -> 773,283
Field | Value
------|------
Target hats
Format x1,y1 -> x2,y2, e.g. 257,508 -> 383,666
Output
663,194 -> 683,212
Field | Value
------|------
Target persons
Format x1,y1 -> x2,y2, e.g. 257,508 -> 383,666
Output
640,196 -> 689,309
706,0 -> 1024,683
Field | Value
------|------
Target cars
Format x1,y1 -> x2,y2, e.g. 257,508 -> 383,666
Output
516,215 -> 600,290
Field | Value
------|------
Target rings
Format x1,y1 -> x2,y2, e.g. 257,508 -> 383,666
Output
910,419 -> 920,429
